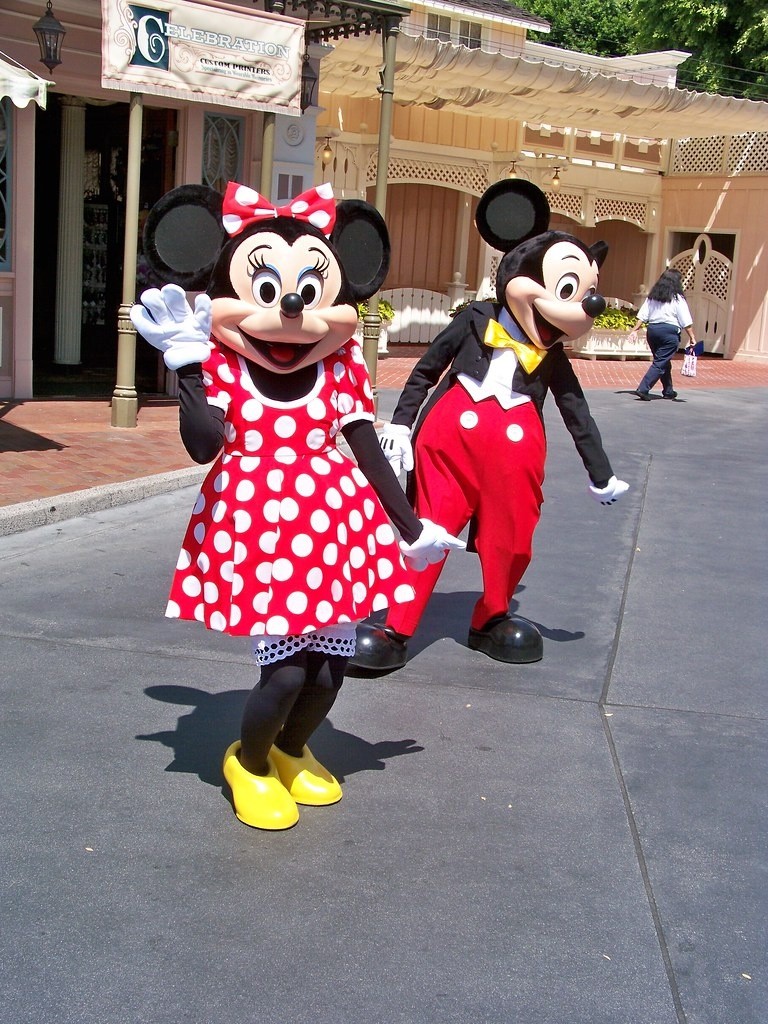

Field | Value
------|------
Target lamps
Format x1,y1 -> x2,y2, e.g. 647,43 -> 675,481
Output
322,135 -> 335,166
551,167 -> 562,194
508,160 -> 518,179
32,0 -> 68,76
300,8 -> 318,114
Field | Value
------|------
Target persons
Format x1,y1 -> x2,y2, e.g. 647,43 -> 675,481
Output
628,269 -> 697,401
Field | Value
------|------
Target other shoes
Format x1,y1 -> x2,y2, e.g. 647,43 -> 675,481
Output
667,390 -> 678,400
636,389 -> 651,400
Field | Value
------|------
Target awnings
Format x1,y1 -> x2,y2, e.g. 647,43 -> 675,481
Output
1,52 -> 55,111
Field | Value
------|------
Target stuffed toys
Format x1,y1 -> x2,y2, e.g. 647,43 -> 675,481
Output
131,181 -> 467,831
350,179 -> 629,669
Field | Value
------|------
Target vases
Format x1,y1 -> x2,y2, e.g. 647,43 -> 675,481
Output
351,320 -> 391,359
571,325 -> 655,361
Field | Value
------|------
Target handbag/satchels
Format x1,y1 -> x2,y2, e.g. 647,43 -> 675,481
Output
682,346 -> 697,377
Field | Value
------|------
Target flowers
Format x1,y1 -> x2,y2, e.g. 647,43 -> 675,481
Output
357,297 -> 394,320
448,294 -> 499,319
594,294 -> 644,327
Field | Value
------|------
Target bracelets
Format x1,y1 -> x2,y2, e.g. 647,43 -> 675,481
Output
690,336 -> 694,339
